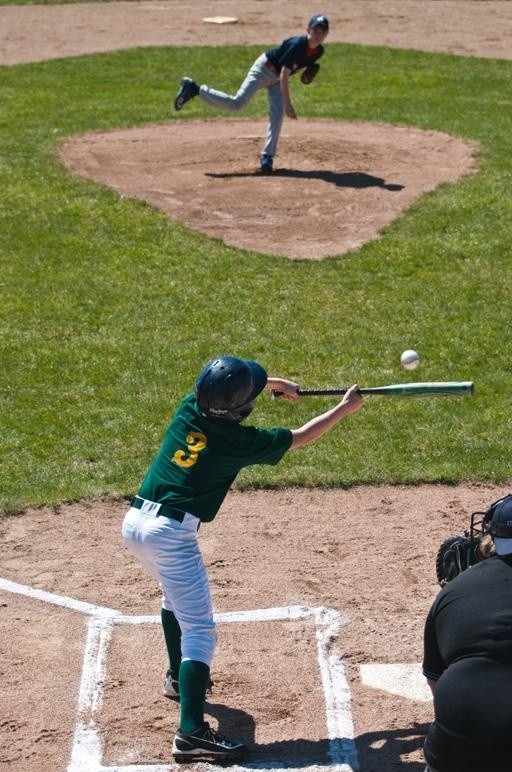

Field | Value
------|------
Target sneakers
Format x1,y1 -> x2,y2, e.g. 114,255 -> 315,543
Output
260,155 -> 273,173
171,723 -> 249,759
174,76 -> 195,111
163,669 -> 214,697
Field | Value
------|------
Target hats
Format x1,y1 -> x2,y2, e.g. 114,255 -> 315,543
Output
308,15 -> 329,31
489,492 -> 512,556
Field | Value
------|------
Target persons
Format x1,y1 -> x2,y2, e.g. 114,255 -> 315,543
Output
121,357 -> 366,763
422,490 -> 512,772
175,15 -> 329,173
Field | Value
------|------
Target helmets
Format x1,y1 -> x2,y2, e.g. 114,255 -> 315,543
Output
193,355 -> 268,423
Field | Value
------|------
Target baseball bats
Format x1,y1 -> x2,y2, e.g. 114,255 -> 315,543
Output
271,381 -> 474,401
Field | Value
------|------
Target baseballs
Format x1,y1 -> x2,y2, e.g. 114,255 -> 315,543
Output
400,349 -> 420,370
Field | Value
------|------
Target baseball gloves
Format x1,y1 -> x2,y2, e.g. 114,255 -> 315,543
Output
436,538 -> 485,588
301,64 -> 320,84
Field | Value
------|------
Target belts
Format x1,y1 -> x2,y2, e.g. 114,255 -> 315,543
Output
130,496 -> 201,532
